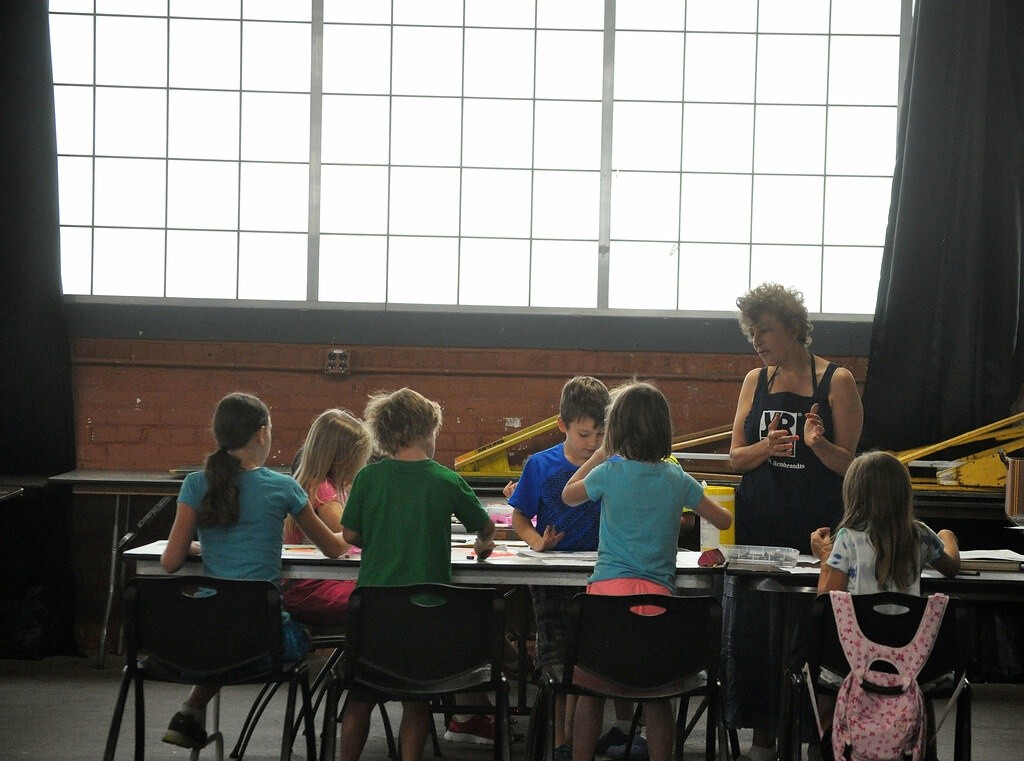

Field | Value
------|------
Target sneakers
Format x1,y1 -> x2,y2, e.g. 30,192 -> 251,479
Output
530,725 -> 650,761
162,712 -> 208,749
443,714 -> 515,745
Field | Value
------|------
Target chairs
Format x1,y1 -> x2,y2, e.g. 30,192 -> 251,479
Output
102,540 -> 1019,761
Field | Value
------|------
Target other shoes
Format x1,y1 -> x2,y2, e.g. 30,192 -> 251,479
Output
746,744 -> 777,761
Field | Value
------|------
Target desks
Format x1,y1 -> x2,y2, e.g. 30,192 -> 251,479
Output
47,467 -> 1024,651
2,477 -> 30,505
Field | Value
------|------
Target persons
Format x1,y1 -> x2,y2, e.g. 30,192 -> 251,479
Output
809,453 -> 960,631
272,407 -> 544,759
562,380 -> 733,761
725,278 -> 864,760
156,390 -> 370,748
508,374 -> 617,760
337,385 -> 497,760
